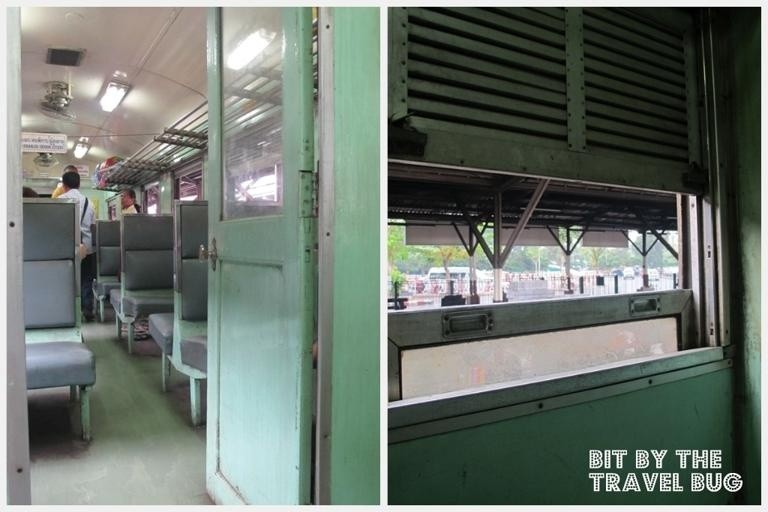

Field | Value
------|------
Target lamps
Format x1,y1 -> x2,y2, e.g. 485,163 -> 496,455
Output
73,143 -> 89,159
100,82 -> 128,112
225,29 -> 272,71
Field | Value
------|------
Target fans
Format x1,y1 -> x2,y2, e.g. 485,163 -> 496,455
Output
33,152 -> 61,171
37,80 -> 76,122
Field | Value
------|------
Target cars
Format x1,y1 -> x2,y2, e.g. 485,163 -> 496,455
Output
578,266 -> 660,282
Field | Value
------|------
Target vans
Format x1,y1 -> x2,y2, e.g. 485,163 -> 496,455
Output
420,266 -> 475,293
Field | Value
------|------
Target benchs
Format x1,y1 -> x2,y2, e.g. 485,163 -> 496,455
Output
88,200 -> 208,427
22,197 -> 96,445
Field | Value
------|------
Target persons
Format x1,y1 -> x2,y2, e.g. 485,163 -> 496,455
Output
22,165 -> 95,322
118,187 -> 142,215
